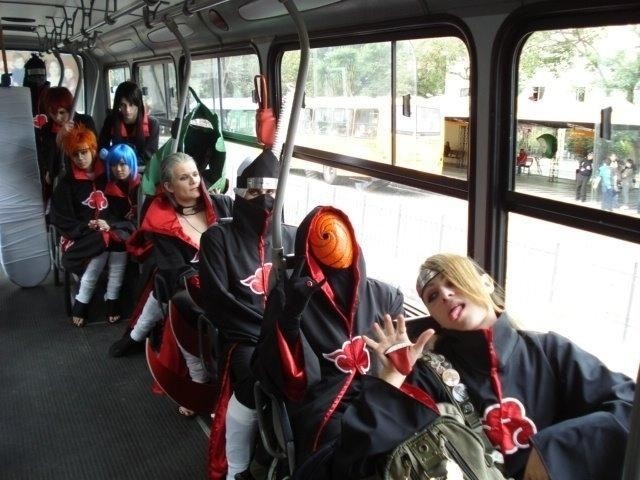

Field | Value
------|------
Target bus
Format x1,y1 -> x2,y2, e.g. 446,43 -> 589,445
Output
188,91 -> 448,186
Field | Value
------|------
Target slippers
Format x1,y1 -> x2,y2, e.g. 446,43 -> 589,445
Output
105,298 -> 122,326
72,299 -> 90,328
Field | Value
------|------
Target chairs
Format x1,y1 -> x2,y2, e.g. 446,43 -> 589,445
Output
47,165 -> 239,325
199,218 -> 639,479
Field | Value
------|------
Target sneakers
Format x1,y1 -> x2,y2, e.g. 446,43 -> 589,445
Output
109,328 -> 145,358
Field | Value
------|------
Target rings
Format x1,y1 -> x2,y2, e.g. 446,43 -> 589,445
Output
304,281 -> 312,288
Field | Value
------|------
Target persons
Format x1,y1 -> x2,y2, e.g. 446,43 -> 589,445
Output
328,252 -> 636,480
444,142 -> 450,157
199,148 -> 299,480
250,205 -> 405,469
575,153 -> 634,211
516,149 -> 527,176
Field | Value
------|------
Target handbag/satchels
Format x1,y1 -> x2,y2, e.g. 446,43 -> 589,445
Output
383,403 -> 517,480
